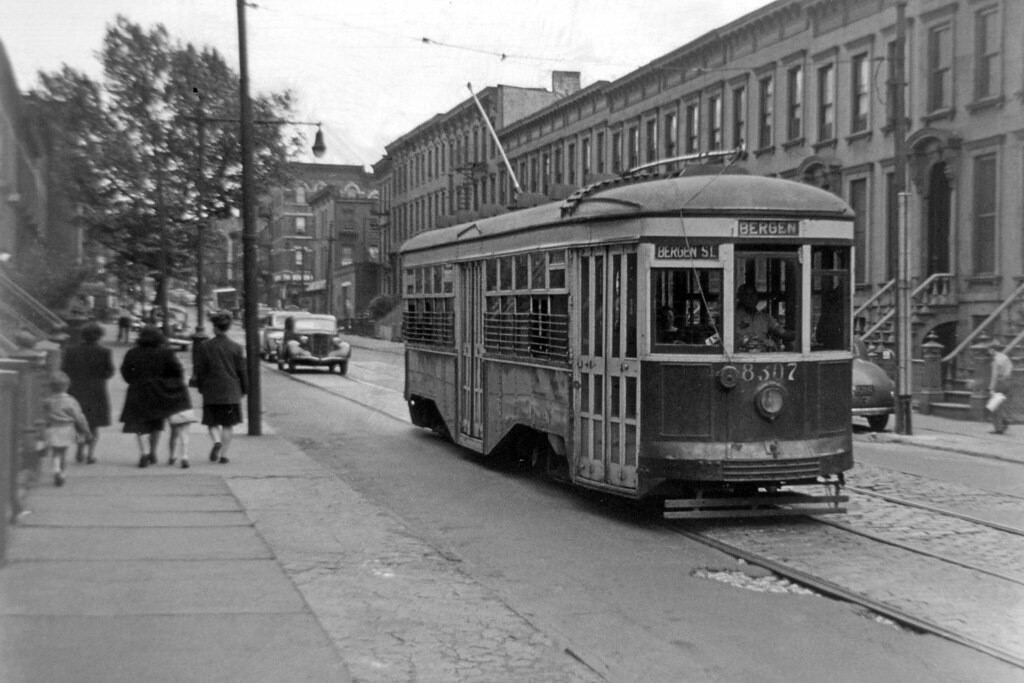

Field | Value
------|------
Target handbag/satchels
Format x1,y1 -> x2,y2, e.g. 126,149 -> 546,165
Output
984,390 -> 1007,413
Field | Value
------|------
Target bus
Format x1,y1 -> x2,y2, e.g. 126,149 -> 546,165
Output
399,163 -> 859,520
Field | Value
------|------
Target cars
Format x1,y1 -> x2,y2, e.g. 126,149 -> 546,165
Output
260,311 -> 352,374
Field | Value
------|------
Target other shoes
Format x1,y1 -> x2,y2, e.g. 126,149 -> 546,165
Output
210,442 -> 221,460
220,458 -> 228,464
86,457 -> 97,464
1002,420 -> 1009,430
76,451 -> 82,460
181,459 -> 189,468
150,453 -> 157,465
989,431 -> 1003,435
140,456 -> 147,467
168,458 -> 174,465
55,472 -> 64,487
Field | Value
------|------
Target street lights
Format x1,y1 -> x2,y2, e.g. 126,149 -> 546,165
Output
240,116 -> 327,438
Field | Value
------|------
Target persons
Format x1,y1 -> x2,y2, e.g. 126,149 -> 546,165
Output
35,369 -> 94,485
60,324 -> 115,464
166,376 -> 196,467
986,341 -> 1013,434
734,282 -> 796,345
195,309 -> 249,464
118,325 -> 184,467
